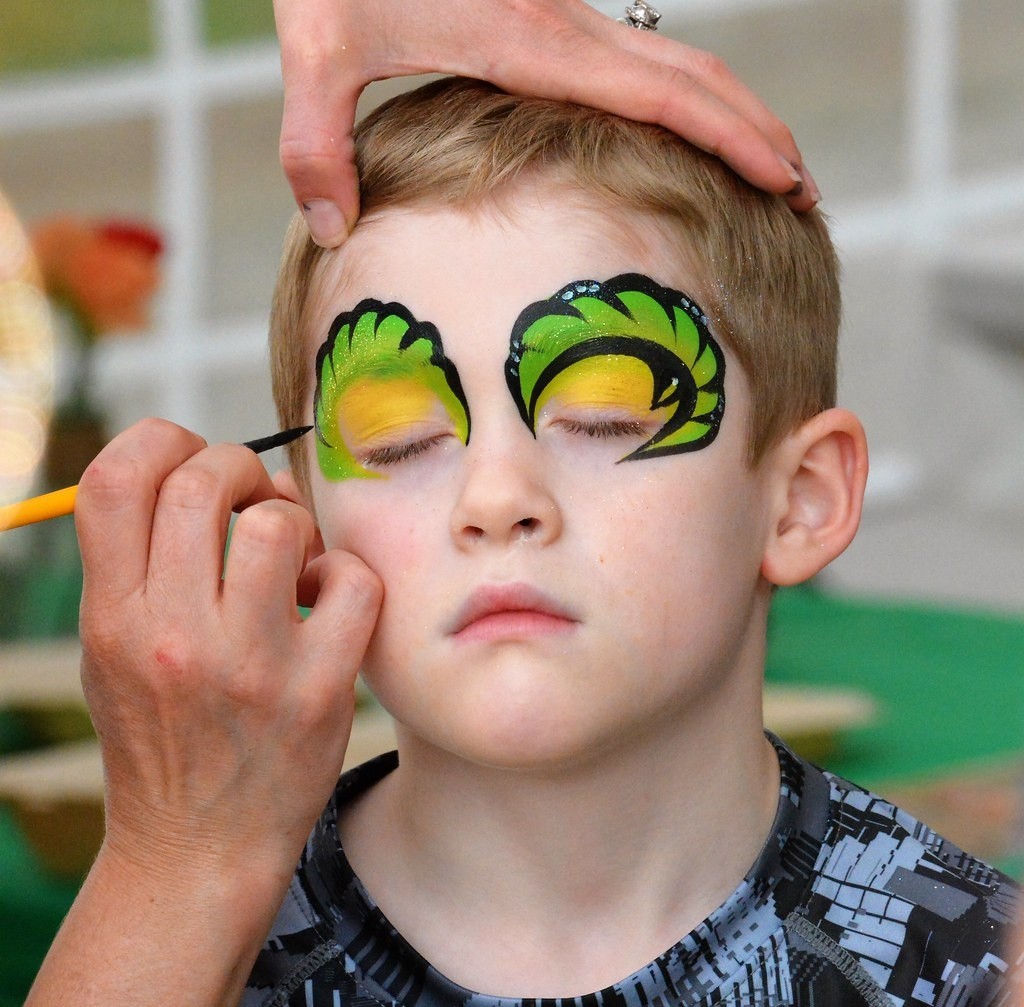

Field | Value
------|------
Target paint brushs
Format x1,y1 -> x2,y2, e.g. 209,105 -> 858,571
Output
0,423 -> 316,533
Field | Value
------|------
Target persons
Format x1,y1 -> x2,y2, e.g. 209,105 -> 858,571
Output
244,77 -> 1024,1007
0,0 -> 823,1007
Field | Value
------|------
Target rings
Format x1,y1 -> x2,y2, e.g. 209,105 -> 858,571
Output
617,0 -> 661,31
